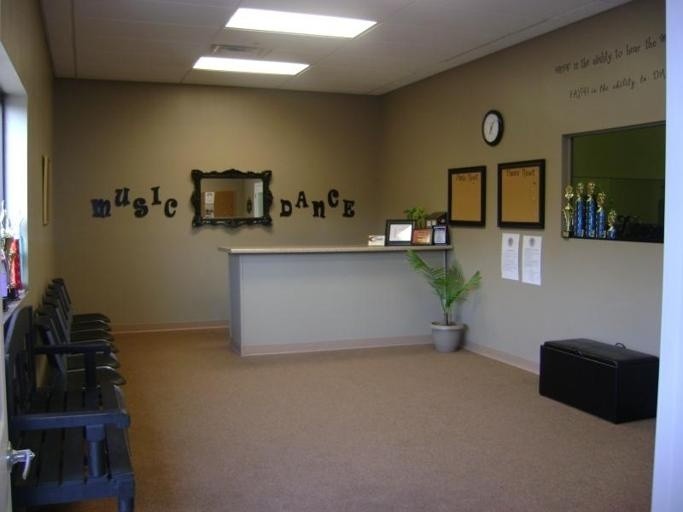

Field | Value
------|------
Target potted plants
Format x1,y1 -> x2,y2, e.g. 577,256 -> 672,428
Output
405,248 -> 481,352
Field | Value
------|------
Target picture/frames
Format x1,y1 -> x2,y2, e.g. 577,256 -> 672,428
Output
411,228 -> 433,246
385,219 -> 414,246
43,155 -> 51,225
448,167 -> 485,226
497,159 -> 544,229
432,225 -> 449,246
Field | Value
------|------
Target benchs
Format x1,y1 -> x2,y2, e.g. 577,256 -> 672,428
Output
540,338 -> 659,424
4,279 -> 135,512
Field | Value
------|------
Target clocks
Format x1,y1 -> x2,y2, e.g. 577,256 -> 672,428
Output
482,110 -> 504,145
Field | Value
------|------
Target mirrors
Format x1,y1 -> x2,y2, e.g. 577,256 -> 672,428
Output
191,169 -> 273,228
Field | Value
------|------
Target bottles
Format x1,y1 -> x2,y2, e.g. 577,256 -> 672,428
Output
563,182 -> 618,240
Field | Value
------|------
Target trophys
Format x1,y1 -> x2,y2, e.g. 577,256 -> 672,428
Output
562,182 -> 617,240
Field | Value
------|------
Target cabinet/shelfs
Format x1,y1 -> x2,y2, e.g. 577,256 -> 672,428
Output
218,244 -> 454,357
